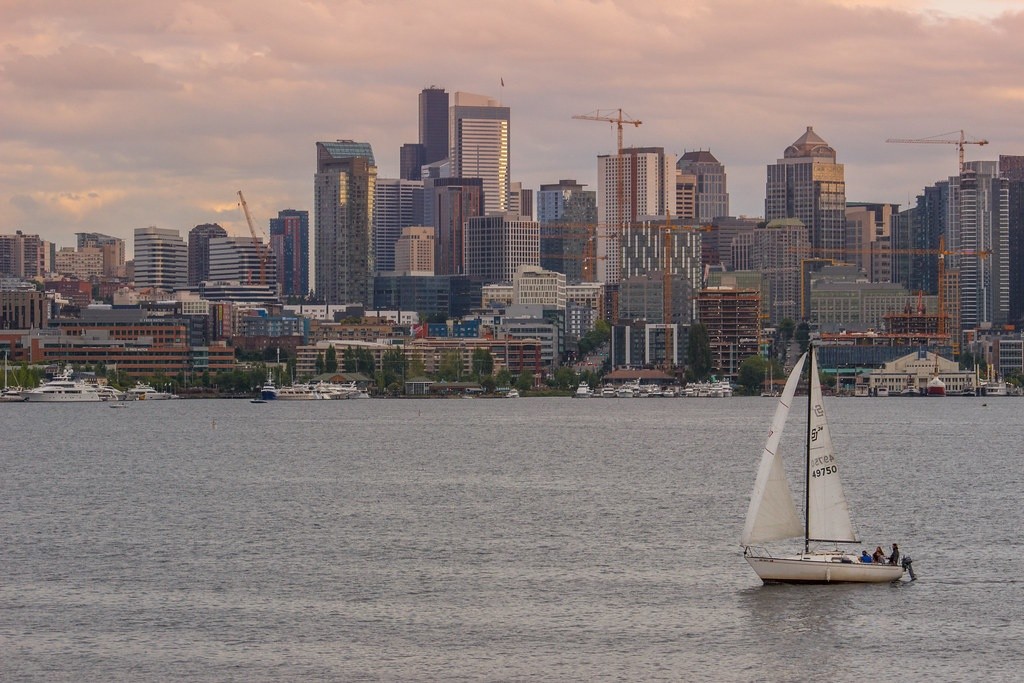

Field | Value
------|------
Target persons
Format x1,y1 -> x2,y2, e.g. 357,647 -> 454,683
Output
872,546 -> 883,560
889,544 -> 899,565
860,551 -> 872,563
876,552 -> 885,566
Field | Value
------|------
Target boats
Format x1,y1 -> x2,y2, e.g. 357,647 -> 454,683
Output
877,381 -> 889,397
960,386 -> 976,396
899,373 -> 921,397
506,388 -> 520,399
0,349 -> 180,402
572,377 -> 733,398
260,369 -> 369,400
854,382 -> 869,397
926,352 -> 947,397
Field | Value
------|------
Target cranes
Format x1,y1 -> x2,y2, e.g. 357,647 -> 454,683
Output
885,128 -> 989,176
570,107 -> 643,281
789,233 -> 994,345
540,210 -> 714,365
236,190 -> 273,283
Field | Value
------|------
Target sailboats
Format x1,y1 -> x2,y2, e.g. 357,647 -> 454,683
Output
761,366 -> 781,397
739,343 -> 920,584
